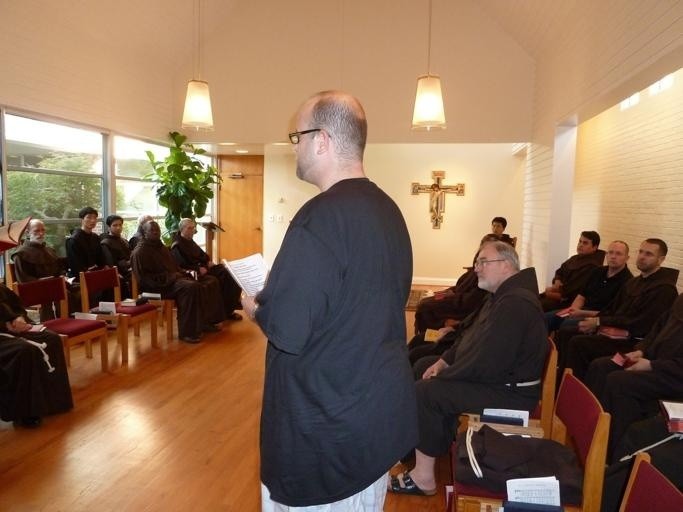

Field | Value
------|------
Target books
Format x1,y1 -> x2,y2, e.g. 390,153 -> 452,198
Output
0,213 -> 31,254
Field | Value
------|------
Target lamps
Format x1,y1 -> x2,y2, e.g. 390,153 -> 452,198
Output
181,0 -> 214,132
412,0 -> 446,131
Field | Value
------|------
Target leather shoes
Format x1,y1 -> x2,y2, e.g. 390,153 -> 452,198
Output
180,312 -> 243,343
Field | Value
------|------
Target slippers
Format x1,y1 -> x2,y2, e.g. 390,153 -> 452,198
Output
387,470 -> 437,496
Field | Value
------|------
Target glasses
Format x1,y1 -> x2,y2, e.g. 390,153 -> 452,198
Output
473,260 -> 506,268
290,129 -> 330,144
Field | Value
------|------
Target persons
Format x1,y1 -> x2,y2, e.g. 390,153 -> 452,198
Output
492,217 -> 514,246
170,218 -> 243,321
561,239 -> 679,381
12,218 -> 81,320
100,215 -> 132,299
0,285 -> 73,427
406,234 -> 501,367
129,214 -> 154,249
65,207 -> 127,324
540,231 -> 607,314
601,415 -> 682,512
386,241 -> 548,496
429,184 -> 443,221
587,292 -> 682,465
130,221 -> 225,343
239,89 -> 419,511
544,241 -> 633,371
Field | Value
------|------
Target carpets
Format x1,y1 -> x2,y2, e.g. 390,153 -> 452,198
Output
405,289 -> 436,311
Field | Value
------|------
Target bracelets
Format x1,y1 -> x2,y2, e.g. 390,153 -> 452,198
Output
249,303 -> 258,320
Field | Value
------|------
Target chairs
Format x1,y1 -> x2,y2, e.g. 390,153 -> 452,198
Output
620,451 -> 683,511
12,275 -> 108,375
5,247 -> 19,294
80,266 -> 159,369
457,336 -> 558,439
132,266 -> 178,342
451,367 -> 611,511
